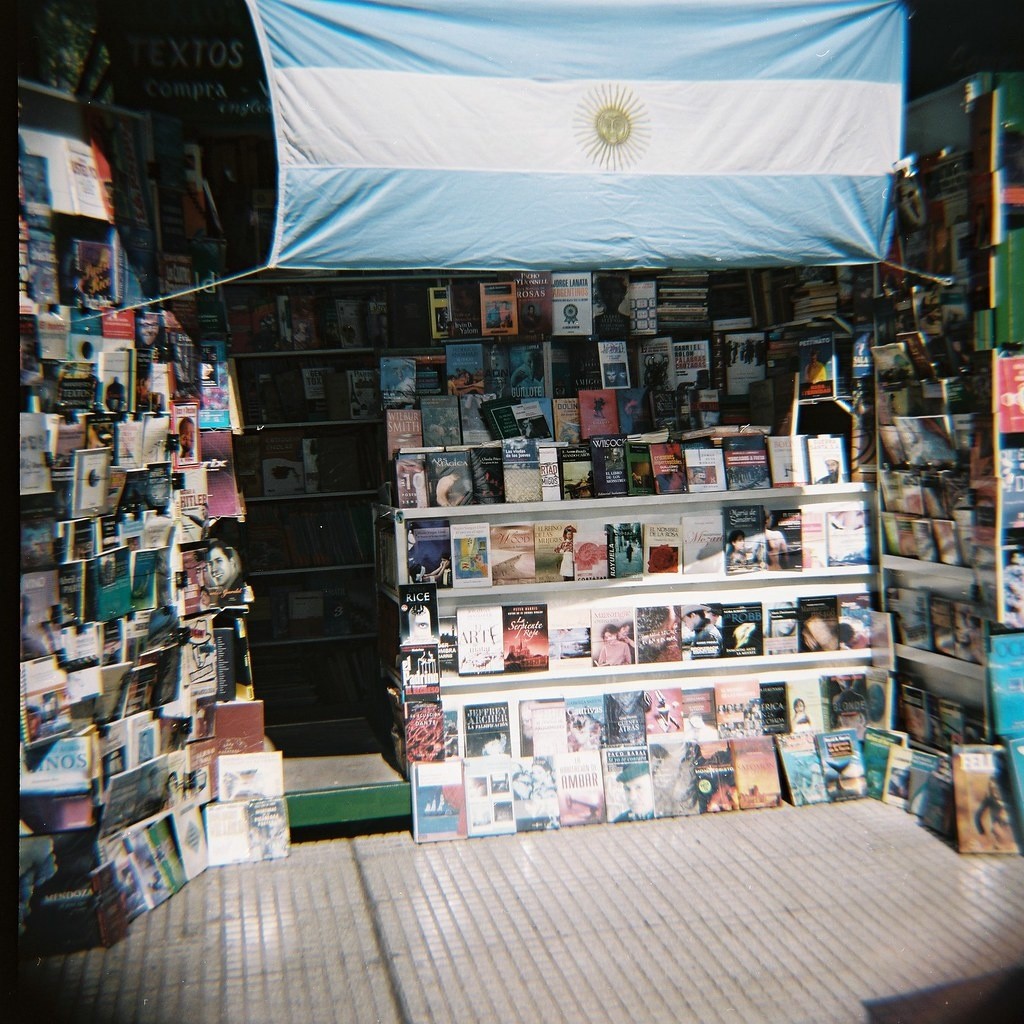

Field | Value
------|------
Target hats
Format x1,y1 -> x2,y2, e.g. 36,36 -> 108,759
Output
616,764 -> 650,783
681,603 -> 711,619
833,690 -> 866,716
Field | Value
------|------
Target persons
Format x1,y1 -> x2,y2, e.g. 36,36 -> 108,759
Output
791,697 -> 815,731
813,455 -> 840,485
204,540 -> 255,609
803,347 -> 828,385
681,603 -> 724,660
618,623 -> 635,663
727,530 -> 766,570
597,620 -> 632,667
612,763 -> 655,825
626,543 -> 634,563
554,526 -> 577,581
485,300 -> 514,328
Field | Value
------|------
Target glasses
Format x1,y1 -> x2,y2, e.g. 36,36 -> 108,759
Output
515,779 -> 532,786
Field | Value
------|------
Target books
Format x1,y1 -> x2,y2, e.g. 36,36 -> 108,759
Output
19,89 -> 1024,963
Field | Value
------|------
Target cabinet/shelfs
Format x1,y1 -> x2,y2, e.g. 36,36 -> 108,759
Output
395,474 -> 871,694
239,341 -> 383,729
871,264 -> 999,743
